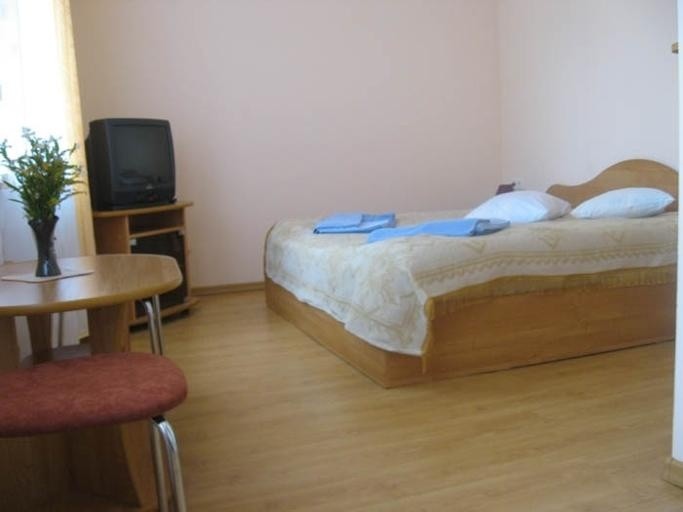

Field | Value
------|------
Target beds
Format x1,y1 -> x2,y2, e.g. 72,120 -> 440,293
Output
263,159 -> 680,389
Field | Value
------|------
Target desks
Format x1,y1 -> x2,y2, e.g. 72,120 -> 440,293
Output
0,253 -> 184,354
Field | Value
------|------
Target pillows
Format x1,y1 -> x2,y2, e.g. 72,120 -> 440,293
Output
464,190 -> 571,223
570,187 -> 676,219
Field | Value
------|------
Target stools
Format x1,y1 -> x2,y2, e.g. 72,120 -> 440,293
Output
0,352 -> 187,512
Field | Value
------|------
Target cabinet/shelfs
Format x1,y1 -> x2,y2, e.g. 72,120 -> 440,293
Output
91,200 -> 200,327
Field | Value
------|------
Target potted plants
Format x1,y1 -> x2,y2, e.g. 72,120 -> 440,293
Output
0,127 -> 88,277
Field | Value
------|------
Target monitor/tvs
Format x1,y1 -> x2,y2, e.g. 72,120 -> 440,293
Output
85,117 -> 175,210
